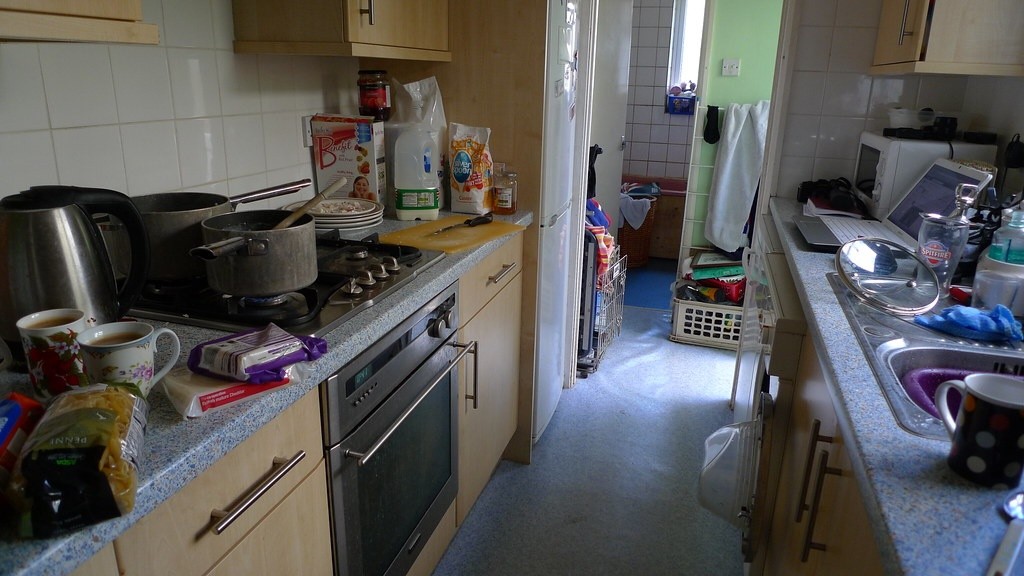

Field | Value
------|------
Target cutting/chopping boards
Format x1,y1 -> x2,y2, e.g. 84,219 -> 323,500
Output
379,215 -> 526,253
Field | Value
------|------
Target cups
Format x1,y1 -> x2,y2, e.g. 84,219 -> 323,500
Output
936,116 -> 957,130
17,308 -> 84,409
917,218 -> 969,298
934,374 -> 1024,489
76,322 -> 180,398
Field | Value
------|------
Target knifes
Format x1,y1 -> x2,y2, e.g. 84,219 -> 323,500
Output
435,214 -> 493,234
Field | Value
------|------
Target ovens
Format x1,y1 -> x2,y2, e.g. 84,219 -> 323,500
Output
322,279 -> 474,576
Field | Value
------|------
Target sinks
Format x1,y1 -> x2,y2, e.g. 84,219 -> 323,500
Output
825,270 -> 1024,441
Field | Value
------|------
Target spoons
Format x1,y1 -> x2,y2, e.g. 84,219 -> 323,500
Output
986,492 -> 1024,576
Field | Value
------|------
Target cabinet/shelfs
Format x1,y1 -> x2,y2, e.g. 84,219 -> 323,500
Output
231,0 -> 452,63
0,0 -> 159,45
455,227 -> 525,532
762,323 -> 888,576
67,385 -> 334,576
867,0 -> 1024,77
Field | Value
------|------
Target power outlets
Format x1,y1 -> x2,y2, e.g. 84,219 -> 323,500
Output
302,116 -> 315,148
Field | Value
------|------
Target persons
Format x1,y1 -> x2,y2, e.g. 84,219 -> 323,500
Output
348,175 -> 376,201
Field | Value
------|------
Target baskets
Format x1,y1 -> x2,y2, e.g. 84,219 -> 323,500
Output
668,281 -> 743,351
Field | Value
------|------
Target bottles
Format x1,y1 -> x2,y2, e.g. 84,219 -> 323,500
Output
991,211 -> 1024,265
394,123 -> 440,220
491,170 -> 518,214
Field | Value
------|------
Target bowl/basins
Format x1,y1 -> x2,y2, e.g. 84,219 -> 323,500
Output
974,245 -> 1024,316
887,108 -> 943,130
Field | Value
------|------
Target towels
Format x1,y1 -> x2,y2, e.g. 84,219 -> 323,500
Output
704,100 -> 771,253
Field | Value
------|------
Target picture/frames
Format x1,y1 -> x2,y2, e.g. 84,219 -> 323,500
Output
691,251 -> 750,269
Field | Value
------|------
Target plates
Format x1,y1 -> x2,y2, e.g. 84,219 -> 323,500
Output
282,198 -> 385,235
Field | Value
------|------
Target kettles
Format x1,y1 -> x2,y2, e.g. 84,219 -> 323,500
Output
0,186 -> 150,361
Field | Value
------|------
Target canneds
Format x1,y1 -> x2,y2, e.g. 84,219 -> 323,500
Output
492,170 -> 518,216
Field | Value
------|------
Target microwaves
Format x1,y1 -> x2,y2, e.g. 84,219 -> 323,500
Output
852,130 -> 998,222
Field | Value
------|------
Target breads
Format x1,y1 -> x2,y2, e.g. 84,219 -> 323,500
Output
166,373 -> 295,414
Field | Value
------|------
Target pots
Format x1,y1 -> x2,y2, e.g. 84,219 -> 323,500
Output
106,178 -> 311,276
189,210 -> 317,295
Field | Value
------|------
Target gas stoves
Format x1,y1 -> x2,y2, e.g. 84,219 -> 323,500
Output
127,229 -> 445,337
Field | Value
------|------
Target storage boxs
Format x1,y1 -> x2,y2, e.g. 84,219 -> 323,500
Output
669,273 -> 763,353
310,112 -> 388,218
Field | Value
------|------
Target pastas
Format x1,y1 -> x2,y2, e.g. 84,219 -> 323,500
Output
48,389 -> 138,516
